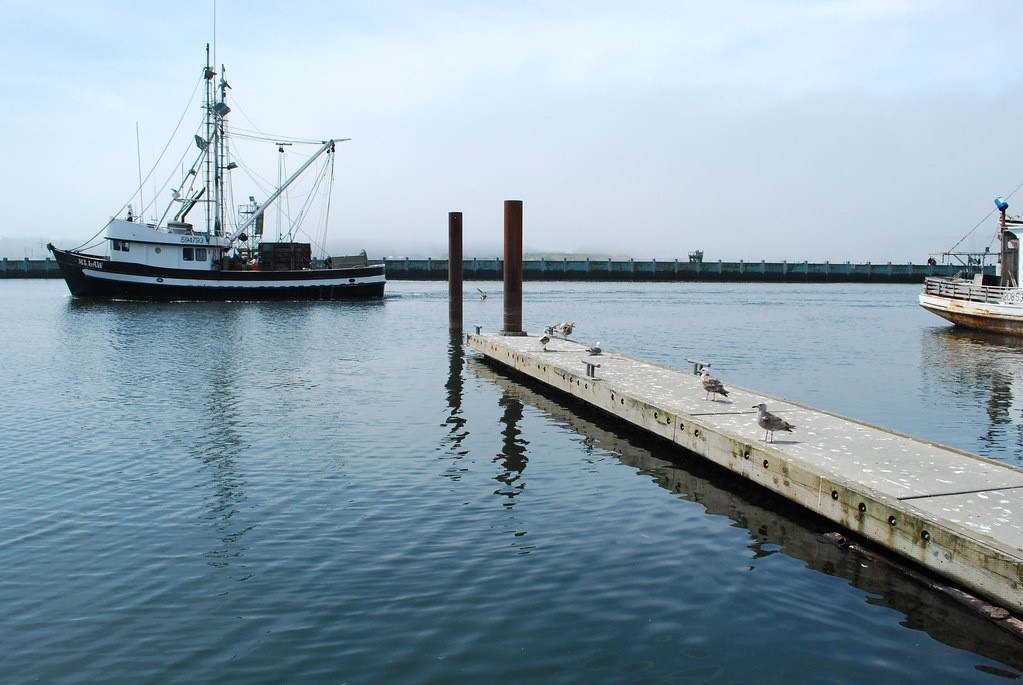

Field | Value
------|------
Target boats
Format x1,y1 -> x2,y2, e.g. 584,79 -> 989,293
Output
919,181 -> 1023,334
44,7 -> 392,303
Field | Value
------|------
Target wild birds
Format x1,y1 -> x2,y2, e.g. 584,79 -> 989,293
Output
539,335 -> 550,350
477,288 -> 487,300
752,402 -> 798,443
695,368 -> 731,401
585,341 -> 602,355
